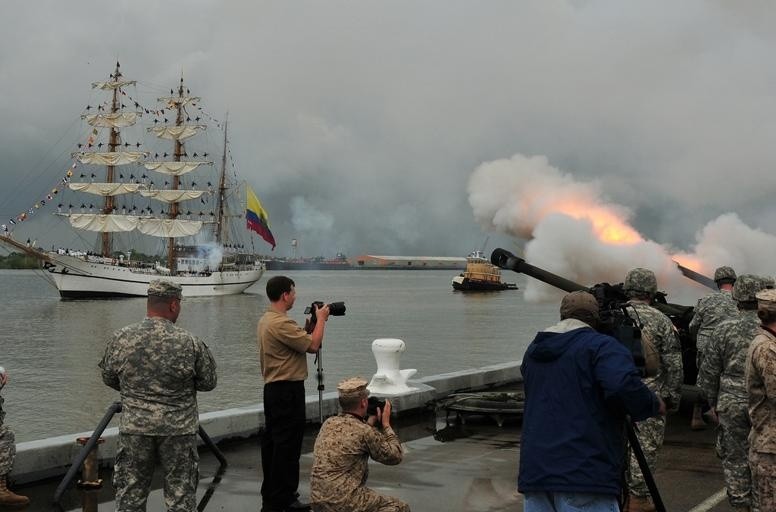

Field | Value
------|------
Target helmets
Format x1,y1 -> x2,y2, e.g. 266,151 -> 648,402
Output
622,266 -> 658,300
730,273 -> 767,304
758,274 -> 775,290
713,265 -> 737,282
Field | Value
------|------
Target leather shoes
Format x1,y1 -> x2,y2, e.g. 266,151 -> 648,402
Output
261,498 -> 315,511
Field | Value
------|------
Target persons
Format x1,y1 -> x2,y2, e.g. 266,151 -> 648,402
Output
258,276 -> 330,512
98,278 -> 218,512
0,367 -> 30,509
28,238 -> 152,269
310,377 -> 411,512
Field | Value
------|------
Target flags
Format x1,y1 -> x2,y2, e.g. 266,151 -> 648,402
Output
246,181 -> 276,251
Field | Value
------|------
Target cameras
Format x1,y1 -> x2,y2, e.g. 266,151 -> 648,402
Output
367,397 -> 392,416
304,301 -> 346,323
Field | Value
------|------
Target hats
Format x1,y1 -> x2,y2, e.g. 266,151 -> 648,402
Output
335,375 -> 371,400
559,290 -> 600,318
754,288 -> 775,314
146,278 -> 187,303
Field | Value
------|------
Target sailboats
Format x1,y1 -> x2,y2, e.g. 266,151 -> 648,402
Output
0,56 -> 282,303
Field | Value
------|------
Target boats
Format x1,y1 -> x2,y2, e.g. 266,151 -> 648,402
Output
263,239 -> 352,271
1,244 -> 776,512
448,234 -> 520,293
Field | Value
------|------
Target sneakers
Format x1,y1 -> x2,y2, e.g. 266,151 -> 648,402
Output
627,492 -> 657,511
